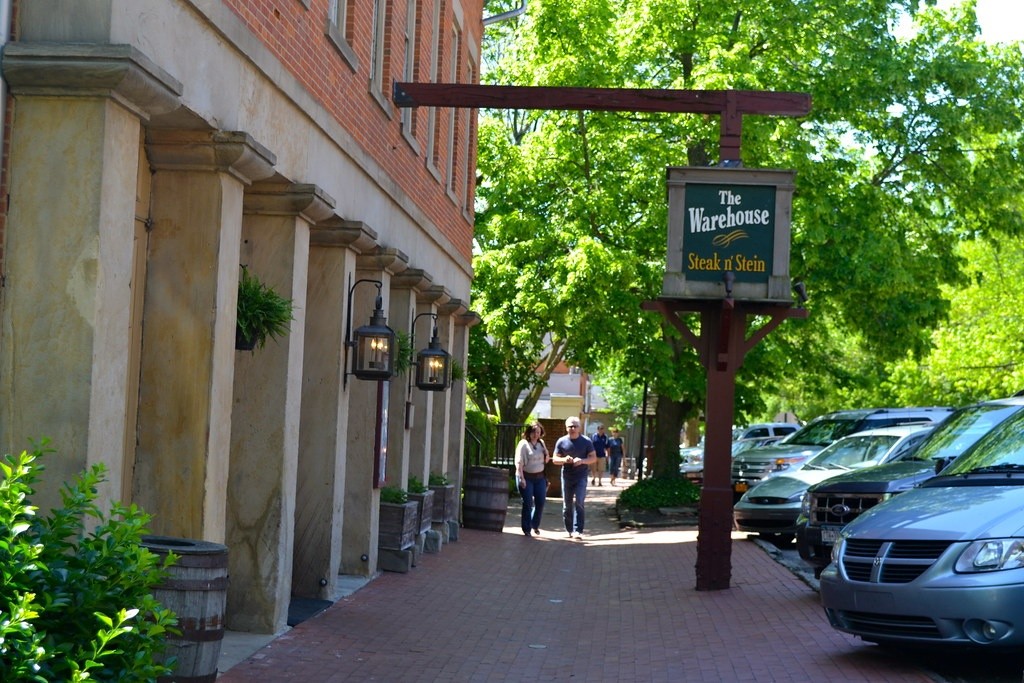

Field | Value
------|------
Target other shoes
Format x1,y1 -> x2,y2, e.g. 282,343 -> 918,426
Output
573,532 -> 581,539
563,530 -> 572,538
534,528 -> 540,535
525,532 -> 531,537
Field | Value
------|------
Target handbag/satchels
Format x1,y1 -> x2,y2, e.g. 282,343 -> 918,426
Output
621,458 -> 629,479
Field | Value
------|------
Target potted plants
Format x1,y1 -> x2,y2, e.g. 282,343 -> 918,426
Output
379,484 -> 419,550
409,476 -> 433,534
428,475 -> 455,524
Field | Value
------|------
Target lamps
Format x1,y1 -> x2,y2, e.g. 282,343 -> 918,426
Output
408,310 -> 450,397
345,271 -> 394,390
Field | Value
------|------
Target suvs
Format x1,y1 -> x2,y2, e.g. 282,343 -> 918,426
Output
793,390 -> 1024,581
730,405 -> 957,501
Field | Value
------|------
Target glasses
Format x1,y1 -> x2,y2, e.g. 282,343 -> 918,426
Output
568,425 -> 578,428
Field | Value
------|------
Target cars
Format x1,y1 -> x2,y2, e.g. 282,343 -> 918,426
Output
817,406 -> 1024,672
731,420 -> 941,551
678,422 -> 801,482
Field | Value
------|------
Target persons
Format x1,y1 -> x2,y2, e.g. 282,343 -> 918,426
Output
552,416 -> 596,538
515,422 -> 549,536
609,429 -> 625,485
589,425 -> 610,486
